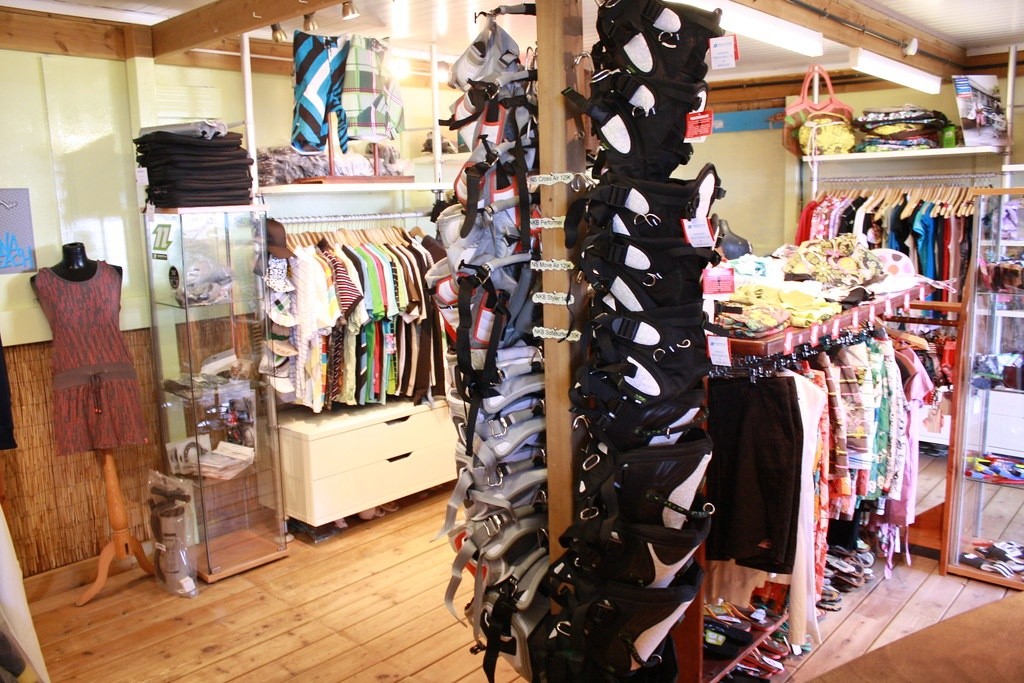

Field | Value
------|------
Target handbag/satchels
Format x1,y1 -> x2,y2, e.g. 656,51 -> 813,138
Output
438,0 -> 726,683
779,65 -> 855,157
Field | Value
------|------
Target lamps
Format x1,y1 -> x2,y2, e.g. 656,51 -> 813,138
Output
847,48 -> 941,94
342,2 -> 360,20
271,23 -> 288,44
303,11 -> 318,32
654,0 -> 826,58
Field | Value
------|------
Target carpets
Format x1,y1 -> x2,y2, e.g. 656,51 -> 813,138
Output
806,591 -> 1024,683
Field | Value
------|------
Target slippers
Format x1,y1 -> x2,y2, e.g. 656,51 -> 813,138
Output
703,537 -> 877,683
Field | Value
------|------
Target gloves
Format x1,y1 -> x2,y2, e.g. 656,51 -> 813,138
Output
960,539 -> 1023,578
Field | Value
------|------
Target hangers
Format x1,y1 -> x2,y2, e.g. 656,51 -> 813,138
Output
706,314 -> 929,384
806,171 -> 1004,222
254,211 -> 447,260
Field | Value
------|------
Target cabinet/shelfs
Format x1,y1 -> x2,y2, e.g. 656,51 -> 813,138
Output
949,190 -> 1024,593
975,164 -> 1024,354
919,383 -> 1024,459
143,204 -> 290,585
252,393 -> 459,529
239,30 -> 457,206
